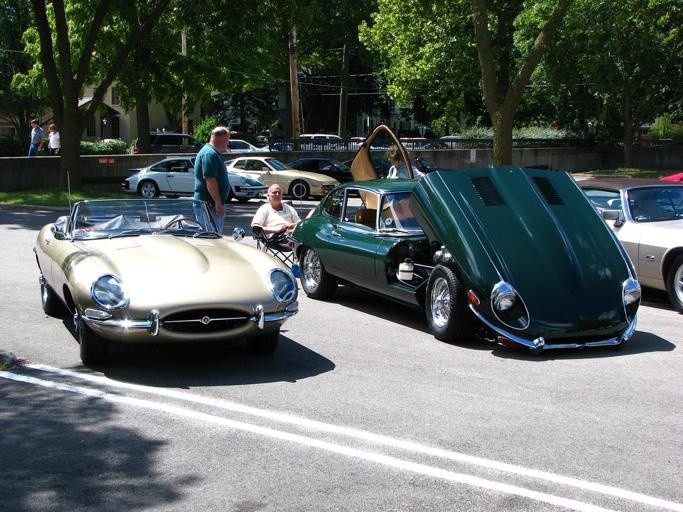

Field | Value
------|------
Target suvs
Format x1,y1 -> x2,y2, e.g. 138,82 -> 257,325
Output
132,131 -> 204,153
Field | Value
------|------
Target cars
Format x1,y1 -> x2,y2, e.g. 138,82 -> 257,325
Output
119,155 -> 396,201
34,173 -> 299,365
290,121 -> 644,354
223,139 -> 293,153
575,178 -> 683,313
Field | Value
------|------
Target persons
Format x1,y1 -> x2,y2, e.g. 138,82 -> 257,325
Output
46,124 -> 60,156
192,125 -> 230,235
27,119 -> 44,156
250,183 -> 301,246
386,144 -> 425,179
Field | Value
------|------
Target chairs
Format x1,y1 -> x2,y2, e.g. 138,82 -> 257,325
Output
597,197 -> 664,219
356,207 -> 378,231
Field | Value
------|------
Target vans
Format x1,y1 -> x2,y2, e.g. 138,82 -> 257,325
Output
300,134 -> 345,150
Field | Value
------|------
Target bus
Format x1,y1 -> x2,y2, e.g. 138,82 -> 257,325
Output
399,138 -> 431,151
